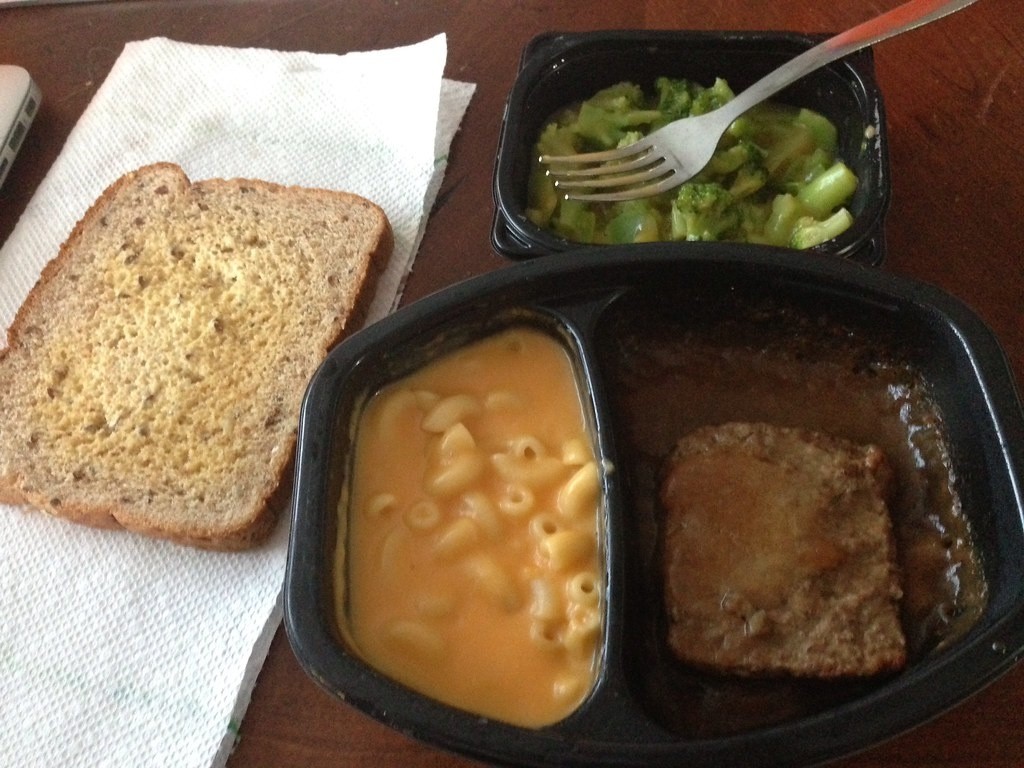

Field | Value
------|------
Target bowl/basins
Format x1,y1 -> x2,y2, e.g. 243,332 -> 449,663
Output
282,28 -> 1024,768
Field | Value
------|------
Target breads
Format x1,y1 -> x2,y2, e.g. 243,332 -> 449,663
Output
0,164 -> 392,548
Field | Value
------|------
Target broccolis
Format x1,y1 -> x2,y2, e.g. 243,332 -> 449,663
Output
529,74 -> 858,248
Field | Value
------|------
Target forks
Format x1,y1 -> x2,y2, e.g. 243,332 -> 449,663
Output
539,0 -> 974,203
